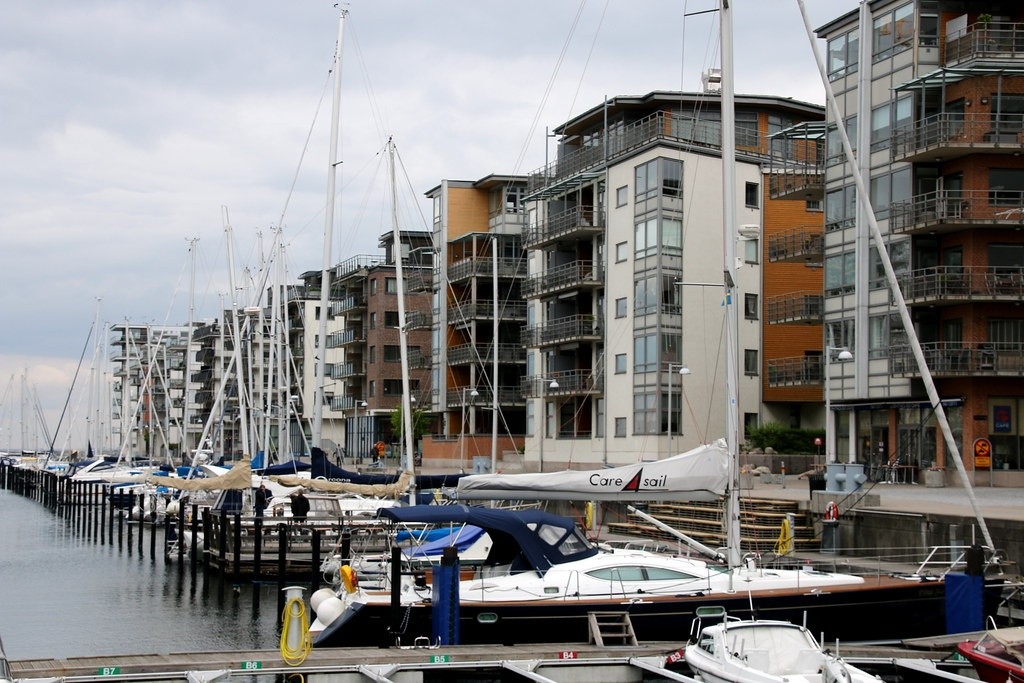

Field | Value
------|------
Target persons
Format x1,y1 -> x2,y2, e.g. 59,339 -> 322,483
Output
255,485 -> 269,526
371,444 -> 380,463
332,444 -> 345,468
290,488 -> 310,543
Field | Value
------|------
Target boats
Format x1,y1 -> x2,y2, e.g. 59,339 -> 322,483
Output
628,604 -> 888,683
956,630 -> 1024,683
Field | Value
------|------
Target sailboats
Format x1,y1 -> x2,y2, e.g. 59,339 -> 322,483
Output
0,1 -> 1018,647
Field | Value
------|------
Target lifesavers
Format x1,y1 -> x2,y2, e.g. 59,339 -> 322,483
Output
825,501 -> 838,521
340,564 -> 356,594
584,501 -> 593,529
